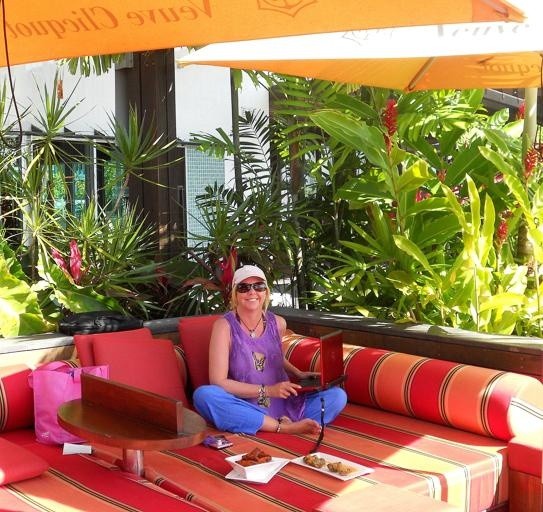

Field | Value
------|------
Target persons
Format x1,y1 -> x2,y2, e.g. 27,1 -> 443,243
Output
192,263 -> 349,436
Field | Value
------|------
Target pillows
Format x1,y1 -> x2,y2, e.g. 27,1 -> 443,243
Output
93,342 -> 190,412
178,316 -> 220,388
75,328 -> 153,369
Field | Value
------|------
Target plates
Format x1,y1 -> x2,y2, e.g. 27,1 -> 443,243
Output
225,457 -> 291,486
290,452 -> 375,482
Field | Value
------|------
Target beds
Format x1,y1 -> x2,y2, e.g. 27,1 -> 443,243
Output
0,402 -> 510,512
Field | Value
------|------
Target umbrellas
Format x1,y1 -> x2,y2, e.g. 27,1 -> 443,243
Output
175,0 -> 542,97
1,0 -> 527,70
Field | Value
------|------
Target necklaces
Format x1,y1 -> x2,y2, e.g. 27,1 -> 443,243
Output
240,317 -> 264,338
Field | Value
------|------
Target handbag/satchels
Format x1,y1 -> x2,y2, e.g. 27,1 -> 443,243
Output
27,361 -> 109,445
58,313 -> 144,336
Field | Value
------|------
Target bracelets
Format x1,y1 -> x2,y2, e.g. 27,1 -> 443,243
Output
257,383 -> 271,408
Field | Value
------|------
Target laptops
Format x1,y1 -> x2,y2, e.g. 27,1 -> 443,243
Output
289,329 -> 348,395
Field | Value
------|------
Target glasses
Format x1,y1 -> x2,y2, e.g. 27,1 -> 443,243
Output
237,282 -> 267,293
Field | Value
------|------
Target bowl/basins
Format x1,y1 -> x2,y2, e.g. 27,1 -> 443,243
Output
225,452 -> 277,477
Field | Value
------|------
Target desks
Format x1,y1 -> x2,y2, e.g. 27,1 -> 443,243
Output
57,373 -> 208,477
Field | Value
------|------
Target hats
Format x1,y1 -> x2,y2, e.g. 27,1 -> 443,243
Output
233,265 -> 267,285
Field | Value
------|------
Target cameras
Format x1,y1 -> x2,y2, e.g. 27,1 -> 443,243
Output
206,437 -> 233,450
